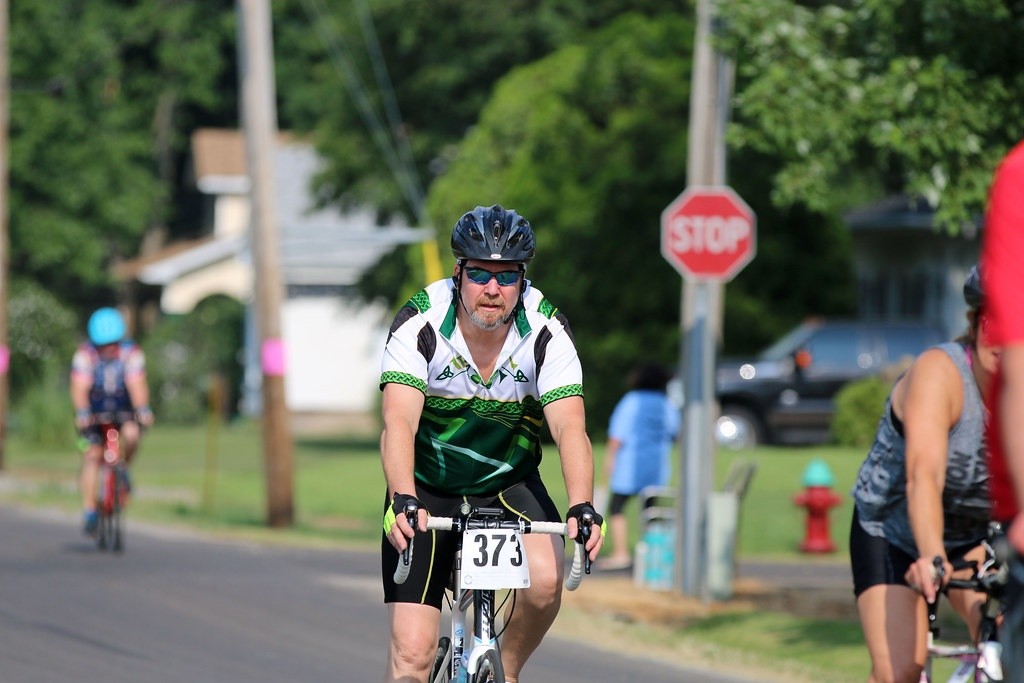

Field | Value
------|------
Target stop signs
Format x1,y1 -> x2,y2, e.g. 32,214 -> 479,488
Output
660,185 -> 758,282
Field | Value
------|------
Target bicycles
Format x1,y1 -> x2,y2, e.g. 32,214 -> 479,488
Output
394,498 -> 592,683
75,412 -> 145,553
919,560 -> 1024,683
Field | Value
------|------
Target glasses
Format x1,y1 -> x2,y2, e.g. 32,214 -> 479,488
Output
462,266 -> 523,286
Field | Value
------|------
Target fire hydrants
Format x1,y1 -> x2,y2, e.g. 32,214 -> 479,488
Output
792,457 -> 842,555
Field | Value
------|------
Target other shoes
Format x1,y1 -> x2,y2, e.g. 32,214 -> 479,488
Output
83,512 -> 96,533
114,464 -> 129,493
594,554 -> 630,570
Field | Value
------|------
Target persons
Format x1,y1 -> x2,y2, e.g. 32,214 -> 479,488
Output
380,205 -> 607,683
850,139 -> 1024,683
71,307 -> 156,533
595,367 -> 682,571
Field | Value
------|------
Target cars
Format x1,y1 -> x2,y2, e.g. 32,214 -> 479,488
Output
712,309 -> 964,446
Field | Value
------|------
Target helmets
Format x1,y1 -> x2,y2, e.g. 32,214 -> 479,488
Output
451,203 -> 536,260
88,307 -> 123,345
963,263 -> 985,311
